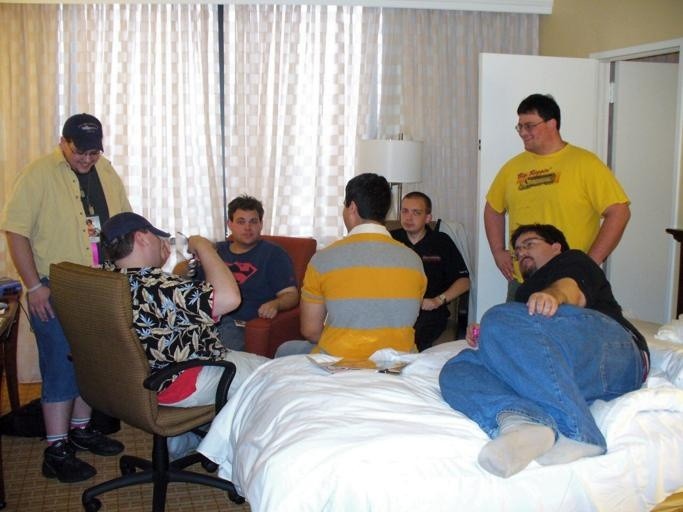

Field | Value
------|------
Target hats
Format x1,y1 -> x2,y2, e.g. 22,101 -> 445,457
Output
101,213 -> 171,242
62,114 -> 105,153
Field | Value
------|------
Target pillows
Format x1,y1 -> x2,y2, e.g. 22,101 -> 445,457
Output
400,336 -> 478,378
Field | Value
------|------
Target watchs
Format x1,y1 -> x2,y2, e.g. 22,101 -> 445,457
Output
437,293 -> 447,305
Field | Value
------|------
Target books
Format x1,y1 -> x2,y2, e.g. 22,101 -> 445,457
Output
305,354 -> 381,375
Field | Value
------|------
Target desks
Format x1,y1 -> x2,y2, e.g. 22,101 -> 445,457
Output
0,287 -> 25,419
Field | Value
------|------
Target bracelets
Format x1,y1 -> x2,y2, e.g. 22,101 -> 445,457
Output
26,282 -> 43,294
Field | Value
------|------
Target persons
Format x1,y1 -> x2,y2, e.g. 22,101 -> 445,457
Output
389,191 -> 470,353
192,195 -> 299,351
438,223 -> 651,478
483,93 -> 632,304
97,212 -> 272,460
272,172 -> 428,360
1,113 -> 134,484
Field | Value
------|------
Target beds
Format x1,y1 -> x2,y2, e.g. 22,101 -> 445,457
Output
194,226 -> 683,512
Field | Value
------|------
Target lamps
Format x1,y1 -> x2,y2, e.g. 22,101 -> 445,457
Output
353,132 -> 424,222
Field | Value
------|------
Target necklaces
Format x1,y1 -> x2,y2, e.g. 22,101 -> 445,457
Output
79,172 -> 95,215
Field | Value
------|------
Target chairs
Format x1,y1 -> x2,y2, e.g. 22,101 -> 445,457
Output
49,261 -> 245,512
382,218 -> 460,349
172,235 -> 317,358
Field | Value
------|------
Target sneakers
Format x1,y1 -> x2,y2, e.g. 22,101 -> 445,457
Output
42,440 -> 97,483
68,428 -> 124,457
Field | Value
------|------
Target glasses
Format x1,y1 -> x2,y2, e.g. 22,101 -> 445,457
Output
67,144 -> 104,157
176,232 -> 198,278
516,120 -> 544,132
514,238 -> 544,260
